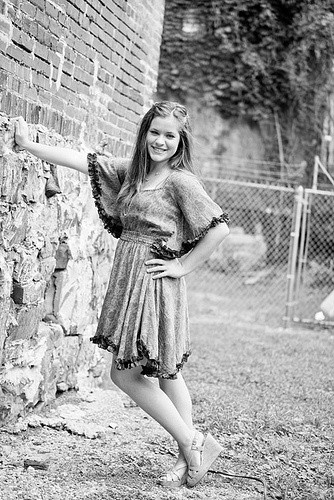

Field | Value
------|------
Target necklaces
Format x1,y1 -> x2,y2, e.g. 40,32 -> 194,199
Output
145,163 -> 170,183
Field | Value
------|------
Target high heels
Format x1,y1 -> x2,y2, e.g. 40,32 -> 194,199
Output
154,451 -> 190,487
180,432 -> 224,486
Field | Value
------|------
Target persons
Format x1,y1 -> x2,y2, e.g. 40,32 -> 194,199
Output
13,102 -> 231,489
